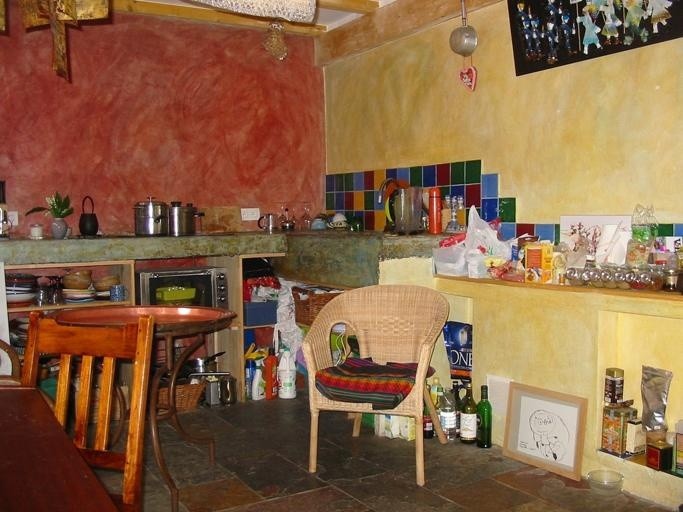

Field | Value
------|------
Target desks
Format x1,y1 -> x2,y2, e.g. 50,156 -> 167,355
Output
46,304 -> 237,511
0,386 -> 119,510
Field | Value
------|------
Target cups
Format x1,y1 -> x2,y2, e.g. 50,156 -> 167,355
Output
109,283 -> 129,302
257,213 -> 279,232
391,187 -> 421,234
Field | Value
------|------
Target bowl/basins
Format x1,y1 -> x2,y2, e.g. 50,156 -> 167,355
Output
585,469 -> 624,495
311,213 -> 347,231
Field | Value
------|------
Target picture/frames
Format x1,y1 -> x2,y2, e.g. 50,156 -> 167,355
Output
500,379 -> 588,483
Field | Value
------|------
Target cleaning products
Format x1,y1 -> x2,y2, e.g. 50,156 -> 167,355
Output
252,356 -> 267,400
263,349 -> 278,400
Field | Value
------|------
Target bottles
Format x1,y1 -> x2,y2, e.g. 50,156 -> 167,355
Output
279,206 -> 311,230
245,343 -> 285,400
663,269 -> 679,291
421,378 -> 492,449
442,194 -> 466,233
563,267 -> 663,291
429,187 -> 442,234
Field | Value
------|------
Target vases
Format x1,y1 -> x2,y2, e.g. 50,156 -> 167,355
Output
48,217 -> 68,240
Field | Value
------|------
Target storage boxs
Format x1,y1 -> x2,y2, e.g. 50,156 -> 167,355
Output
243,299 -> 277,328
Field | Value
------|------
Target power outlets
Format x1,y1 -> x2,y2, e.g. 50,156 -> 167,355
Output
6,211 -> 18,226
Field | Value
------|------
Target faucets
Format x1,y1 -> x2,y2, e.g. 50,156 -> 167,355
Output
377,178 -> 401,204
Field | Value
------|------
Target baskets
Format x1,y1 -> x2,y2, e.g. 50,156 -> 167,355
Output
291,285 -> 342,327
158,382 -> 205,412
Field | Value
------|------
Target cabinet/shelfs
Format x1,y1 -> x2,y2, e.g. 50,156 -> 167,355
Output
207,253 -> 289,404
3,258 -> 138,427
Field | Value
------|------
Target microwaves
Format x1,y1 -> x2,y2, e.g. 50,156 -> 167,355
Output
135,265 -> 227,310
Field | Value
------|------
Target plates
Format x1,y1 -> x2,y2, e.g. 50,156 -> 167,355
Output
5,270 -> 122,306
11,316 -> 33,339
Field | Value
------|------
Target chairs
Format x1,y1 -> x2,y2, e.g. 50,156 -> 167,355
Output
19,310 -> 154,510
299,283 -> 452,487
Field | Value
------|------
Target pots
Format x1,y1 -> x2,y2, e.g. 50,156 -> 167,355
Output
132,196 -> 168,236
193,350 -> 227,372
168,201 -> 204,238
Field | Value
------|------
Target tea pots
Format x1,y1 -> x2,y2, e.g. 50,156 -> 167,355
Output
220,375 -> 239,406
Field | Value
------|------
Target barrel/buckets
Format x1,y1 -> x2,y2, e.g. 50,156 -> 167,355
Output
277,348 -> 298,399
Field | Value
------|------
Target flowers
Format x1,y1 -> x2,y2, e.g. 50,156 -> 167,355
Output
24,189 -> 75,219
